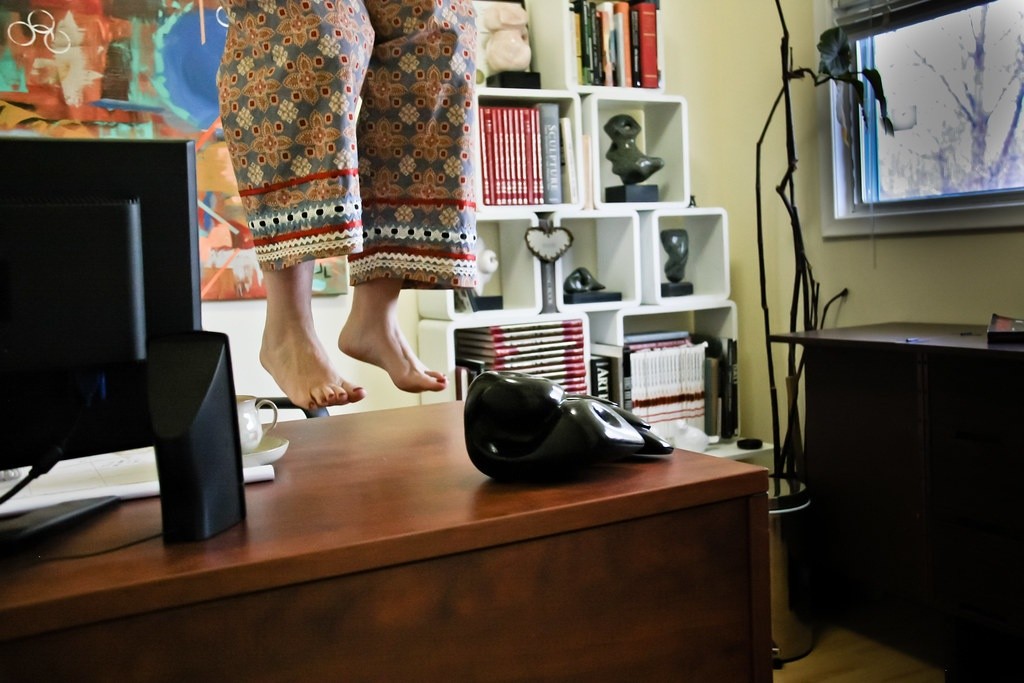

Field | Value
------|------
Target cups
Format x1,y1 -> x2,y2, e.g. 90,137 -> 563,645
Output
236,395 -> 278,454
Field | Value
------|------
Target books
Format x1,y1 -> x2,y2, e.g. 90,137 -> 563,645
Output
569,0 -> 661,88
590,331 -> 738,439
456,319 -> 587,400
478,101 -> 579,206
987,312 -> 1023,345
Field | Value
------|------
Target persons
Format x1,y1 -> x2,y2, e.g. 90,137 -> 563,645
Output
216,0 -> 478,411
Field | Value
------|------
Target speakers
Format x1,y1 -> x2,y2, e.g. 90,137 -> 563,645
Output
146,329 -> 246,544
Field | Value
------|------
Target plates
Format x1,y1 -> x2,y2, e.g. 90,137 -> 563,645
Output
239,434 -> 289,467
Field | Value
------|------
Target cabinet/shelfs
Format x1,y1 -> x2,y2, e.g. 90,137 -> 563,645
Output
0,402 -> 775,682
416,0 -> 782,475
766,321 -> 1024,683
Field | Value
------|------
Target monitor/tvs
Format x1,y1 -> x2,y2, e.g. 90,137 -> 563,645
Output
0,133 -> 203,551
